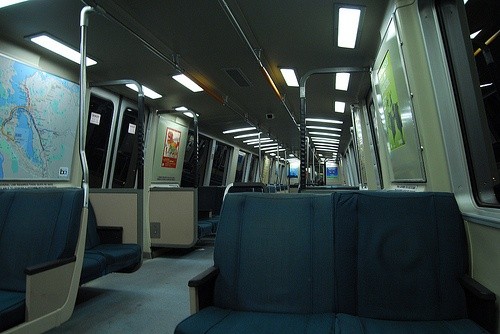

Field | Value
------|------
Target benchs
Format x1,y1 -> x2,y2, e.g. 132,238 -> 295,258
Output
0,176 -> 500,332
